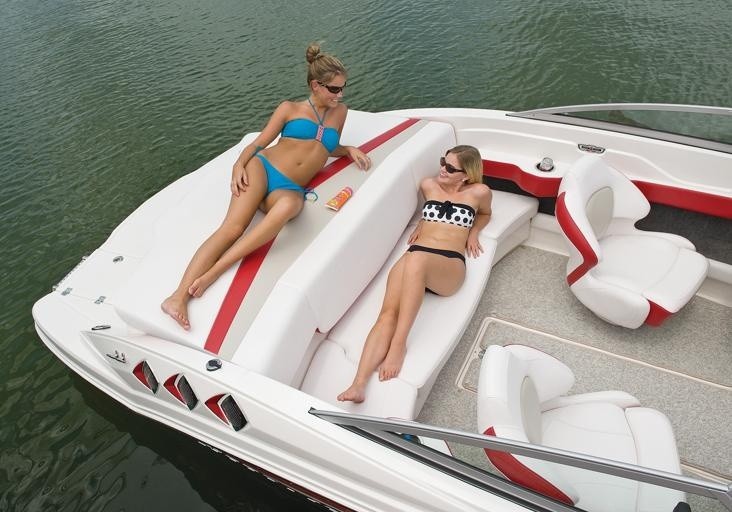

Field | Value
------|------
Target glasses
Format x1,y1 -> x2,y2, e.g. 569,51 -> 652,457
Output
439,157 -> 464,173
317,81 -> 346,94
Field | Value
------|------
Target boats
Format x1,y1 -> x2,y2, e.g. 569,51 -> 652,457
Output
30,102 -> 732,511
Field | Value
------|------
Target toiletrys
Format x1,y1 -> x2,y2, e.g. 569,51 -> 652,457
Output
325,186 -> 353,211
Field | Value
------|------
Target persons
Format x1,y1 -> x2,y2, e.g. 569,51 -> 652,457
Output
161,46 -> 372,331
338,145 -> 492,404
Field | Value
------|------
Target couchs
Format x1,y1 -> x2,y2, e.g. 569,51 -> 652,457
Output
232,121 -> 539,424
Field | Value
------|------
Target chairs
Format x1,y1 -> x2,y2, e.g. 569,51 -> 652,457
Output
555,153 -> 710,329
476,344 -> 687,512
